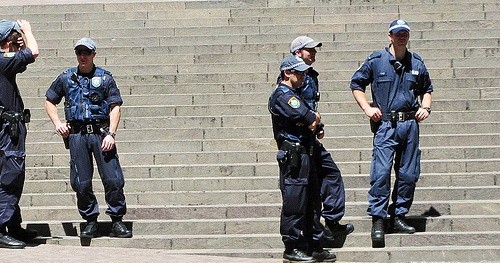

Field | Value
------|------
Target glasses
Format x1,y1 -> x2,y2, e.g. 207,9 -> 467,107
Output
74,50 -> 94,56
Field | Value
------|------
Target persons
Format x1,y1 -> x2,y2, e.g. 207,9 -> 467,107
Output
350,18 -> 434,243
44,37 -> 133,239
275,35 -> 355,239
268,55 -> 337,263
0,19 -> 40,248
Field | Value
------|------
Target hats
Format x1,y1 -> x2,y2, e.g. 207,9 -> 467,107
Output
290,36 -> 322,53
279,57 -> 314,73
388,19 -> 410,35
74,38 -> 97,52
0,20 -> 21,42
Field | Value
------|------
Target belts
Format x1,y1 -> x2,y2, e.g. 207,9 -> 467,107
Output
280,145 -> 315,154
5,113 -> 24,122
69,123 -> 108,134
382,111 -> 416,122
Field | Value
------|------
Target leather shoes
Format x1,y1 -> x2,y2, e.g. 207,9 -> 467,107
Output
307,245 -> 337,262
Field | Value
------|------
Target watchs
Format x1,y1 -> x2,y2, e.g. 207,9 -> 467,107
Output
108,131 -> 116,138
422,107 -> 432,114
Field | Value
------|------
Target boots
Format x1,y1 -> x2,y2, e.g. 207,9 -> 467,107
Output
110,215 -> 132,238
80,216 -> 101,239
0,230 -> 25,249
324,219 -> 354,238
304,228 -> 343,247
371,215 -> 385,242
7,224 -> 38,241
389,215 -> 415,234
282,244 -> 318,263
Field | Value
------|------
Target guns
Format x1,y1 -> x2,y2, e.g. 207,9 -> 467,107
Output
284,138 -> 300,171
2,111 -> 19,141
